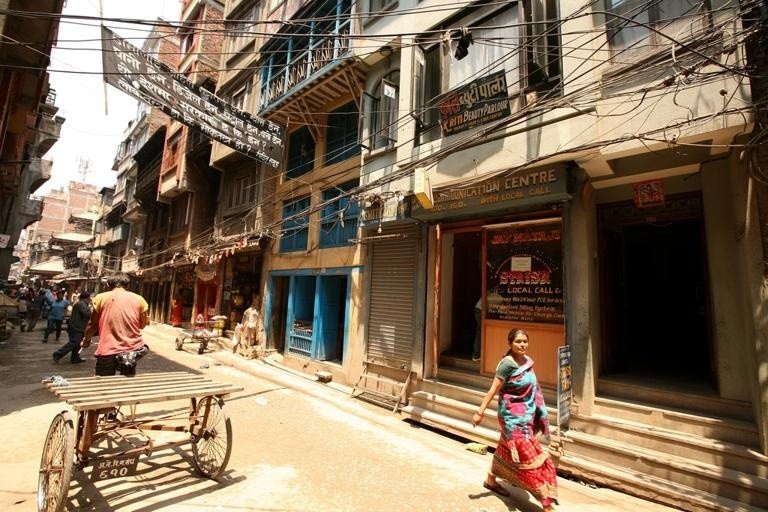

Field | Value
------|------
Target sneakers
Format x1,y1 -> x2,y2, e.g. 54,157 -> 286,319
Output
51,353 -> 86,364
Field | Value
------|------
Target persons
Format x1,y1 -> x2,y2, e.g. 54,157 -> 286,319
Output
472,327 -> 561,512
6,285 -> 92,363
80,271 -> 149,421
170,292 -> 187,326
471,287 -> 507,361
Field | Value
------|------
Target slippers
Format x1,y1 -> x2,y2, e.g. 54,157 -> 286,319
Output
482,480 -> 510,498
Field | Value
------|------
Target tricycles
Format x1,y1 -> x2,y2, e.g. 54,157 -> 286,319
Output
37,340 -> 244,512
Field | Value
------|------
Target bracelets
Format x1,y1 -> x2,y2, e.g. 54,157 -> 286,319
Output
476,411 -> 484,416
